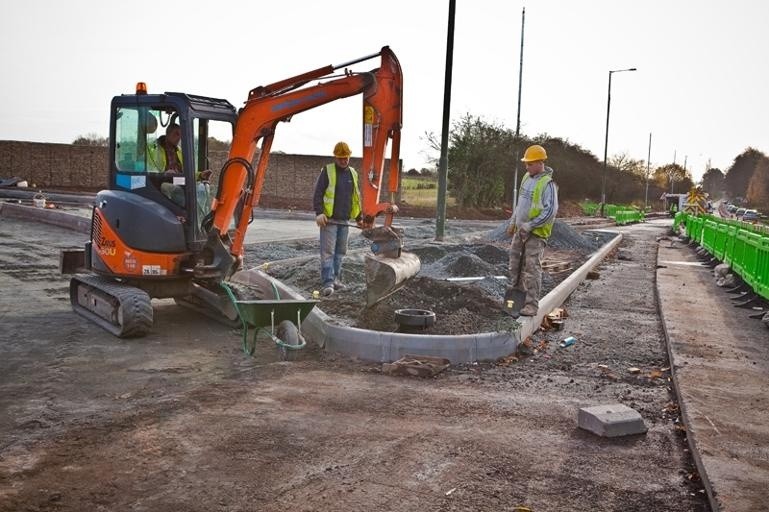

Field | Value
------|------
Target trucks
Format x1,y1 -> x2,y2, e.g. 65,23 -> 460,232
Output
664,192 -> 715,217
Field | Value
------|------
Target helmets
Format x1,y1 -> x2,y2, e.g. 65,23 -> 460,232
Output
520,145 -> 547,162
334,142 -> 351,158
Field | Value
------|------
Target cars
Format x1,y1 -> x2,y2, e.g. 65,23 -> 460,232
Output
719,198 -> 761,224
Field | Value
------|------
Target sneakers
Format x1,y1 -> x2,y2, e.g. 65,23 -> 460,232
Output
334,281 -> 345,289
324,288 -> 333,297
519,304 -> 537,315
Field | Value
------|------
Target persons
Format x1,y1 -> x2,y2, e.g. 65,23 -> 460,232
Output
313,141 -> 363,297
147,124 -> 212,182
506,145 -> 560,317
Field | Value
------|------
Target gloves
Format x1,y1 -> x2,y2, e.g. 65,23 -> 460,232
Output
316,214 -> 327,226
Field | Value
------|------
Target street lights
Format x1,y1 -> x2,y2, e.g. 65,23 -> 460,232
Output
598,67 -> 637,219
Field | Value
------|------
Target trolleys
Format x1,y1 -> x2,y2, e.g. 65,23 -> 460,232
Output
216,265 -> 325,356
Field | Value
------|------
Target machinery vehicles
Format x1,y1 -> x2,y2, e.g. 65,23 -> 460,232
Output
55,46 -> 421,341
